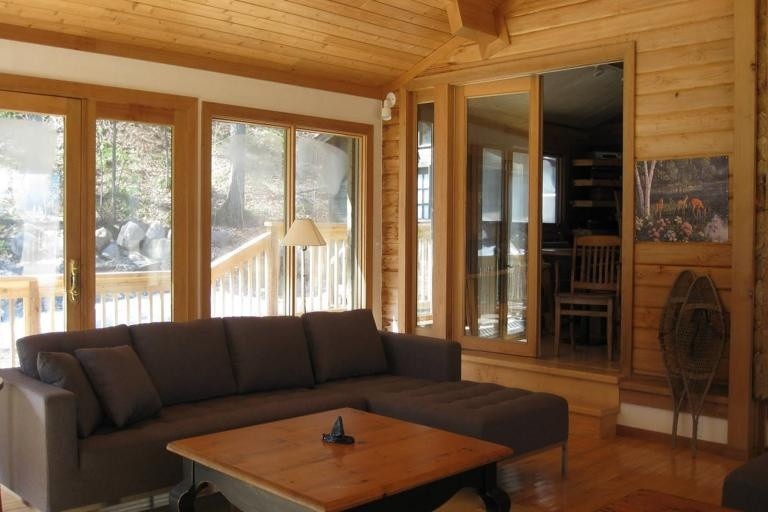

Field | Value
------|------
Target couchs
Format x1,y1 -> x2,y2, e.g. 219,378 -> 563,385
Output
722,447 -> 768,512
367,381 -> 569,482
0,309 -> 461,512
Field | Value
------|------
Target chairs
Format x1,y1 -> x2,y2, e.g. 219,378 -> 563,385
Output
553,236 -> 621,360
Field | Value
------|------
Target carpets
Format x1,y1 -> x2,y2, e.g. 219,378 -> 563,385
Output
599,488 -> 744,512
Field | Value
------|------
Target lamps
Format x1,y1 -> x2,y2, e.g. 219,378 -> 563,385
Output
282,220 -> 327,314
381,92 -> 396,121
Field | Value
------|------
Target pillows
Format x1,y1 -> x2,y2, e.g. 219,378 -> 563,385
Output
36,352 -> 101,439
75,345 -> 162,429
